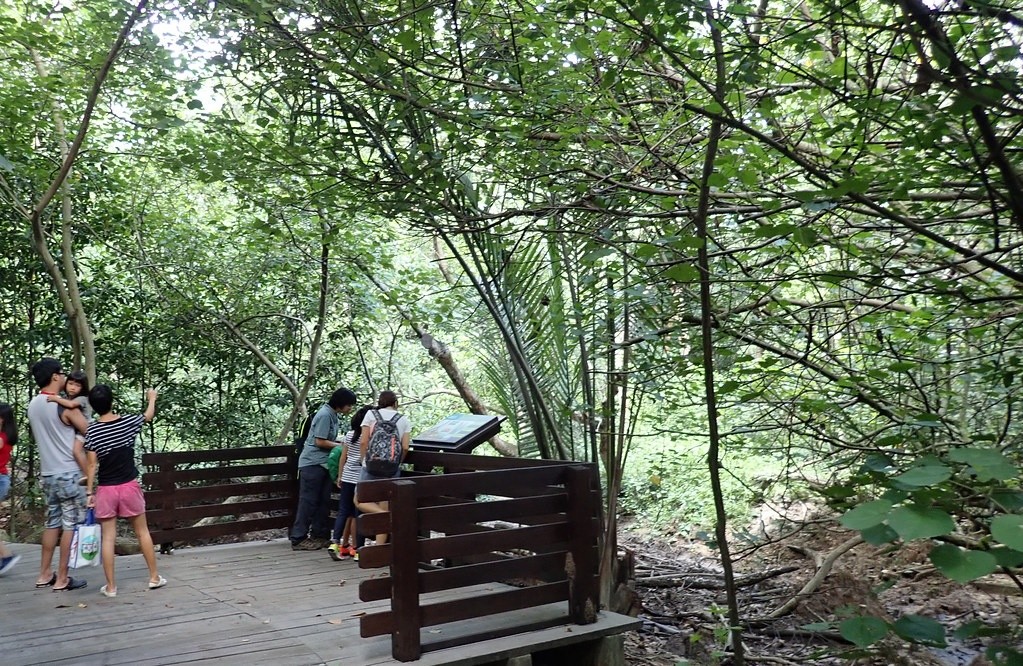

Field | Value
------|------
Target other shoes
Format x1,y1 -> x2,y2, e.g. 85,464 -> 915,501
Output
0,555 -> 19,574
338,545 -> 349,554
349,547 -> 358,556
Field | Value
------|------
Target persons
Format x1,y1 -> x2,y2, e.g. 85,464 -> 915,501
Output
26,357 -> 93,592
0,402 -> 21,574
328,391 -> 411,561
289,387 -> 357,550
82,384 -> 166,597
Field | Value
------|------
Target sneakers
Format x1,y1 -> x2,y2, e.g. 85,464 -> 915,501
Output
310,539 -> 335,548
327,544 -> 343,561
353,553 -> 359,561
291,537 -> 321,551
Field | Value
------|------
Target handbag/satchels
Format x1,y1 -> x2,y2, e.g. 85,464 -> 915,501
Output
67,508 -> 102,569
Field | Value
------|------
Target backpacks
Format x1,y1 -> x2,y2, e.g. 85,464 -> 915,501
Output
328,445 -> 344,480
364,409 -> 404,478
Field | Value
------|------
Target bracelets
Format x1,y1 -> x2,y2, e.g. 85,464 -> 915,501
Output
86,494 -> 92,496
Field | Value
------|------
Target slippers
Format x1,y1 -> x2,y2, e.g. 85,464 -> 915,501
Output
100,584 -> 116,597
148,575 -> 166,588
35,572 -> 57,588
52,576 -> 88,593
